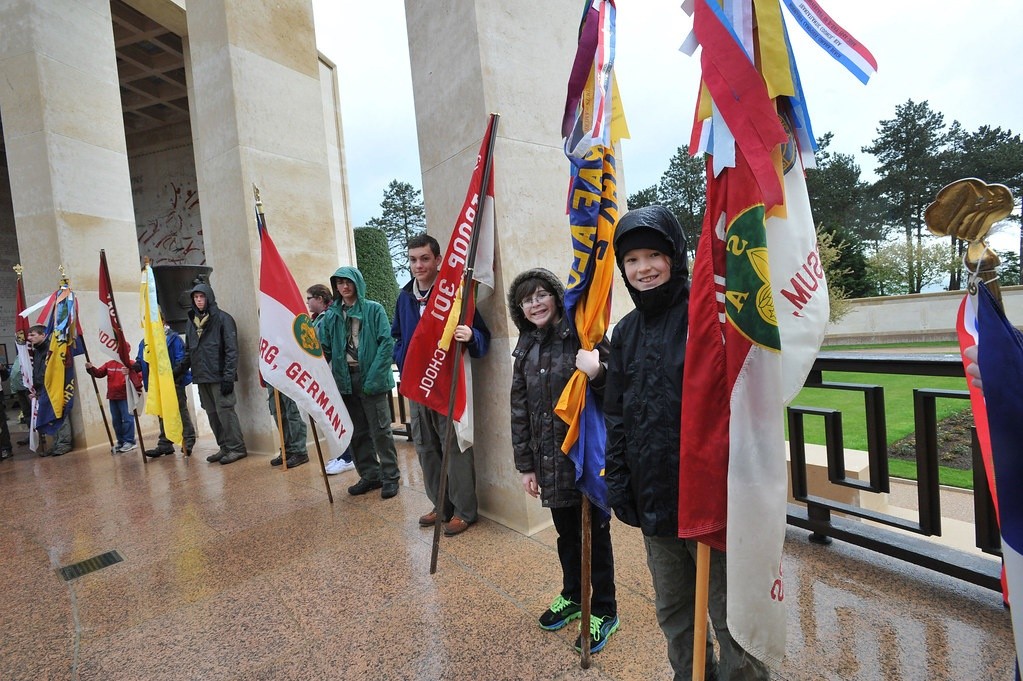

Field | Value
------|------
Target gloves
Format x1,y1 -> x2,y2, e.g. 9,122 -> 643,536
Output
220,381 -> 234,397
177,362 -> 187,375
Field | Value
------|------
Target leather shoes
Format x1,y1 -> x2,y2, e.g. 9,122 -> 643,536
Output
419,511 -> 437,527
442,515 -> 472,534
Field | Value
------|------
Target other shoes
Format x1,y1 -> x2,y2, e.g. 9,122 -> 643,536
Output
181,445 -> 193,456
1,446 -> 14,460
145,445 -> 176,457
39,444 -> 74,456
17,434 -> 32,445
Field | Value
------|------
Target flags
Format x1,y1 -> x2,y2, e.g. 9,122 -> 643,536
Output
135,263 -> 185,451
14,276 -> 86,452
256,222 -> 357,463
958,288 -> 1023,611
679,0 -> 881,681
98,254 -> 145,417
399,121 -> 498,452
555,0 -> 631,519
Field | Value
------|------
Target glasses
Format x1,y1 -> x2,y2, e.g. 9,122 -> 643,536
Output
519,292 -> 554,309
337,280 -> 354,286
306,296 -> 315,301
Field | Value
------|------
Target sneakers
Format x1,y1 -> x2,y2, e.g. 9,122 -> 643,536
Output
270,451 -> 310,468
575,612 -> 620,655
110,442 -> 138,452
381,481 -> 400,498
321,457 -> 356,475
207,449 -> 248,464
539,595 -> 582,631
348,479 -> 383,495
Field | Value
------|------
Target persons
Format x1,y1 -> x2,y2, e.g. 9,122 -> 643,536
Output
85,343 -> 143,452
0,363 -> 14,461
172,283 -> 247,465
9,353 -> 33,445
129,302 -> 196,457
390,234 -> 492,537
306,266 -> 401,498
27,324 -> 72,457
956,281 -> 1023,680
509,266 -> 622,655
602,204 -> 769,681
